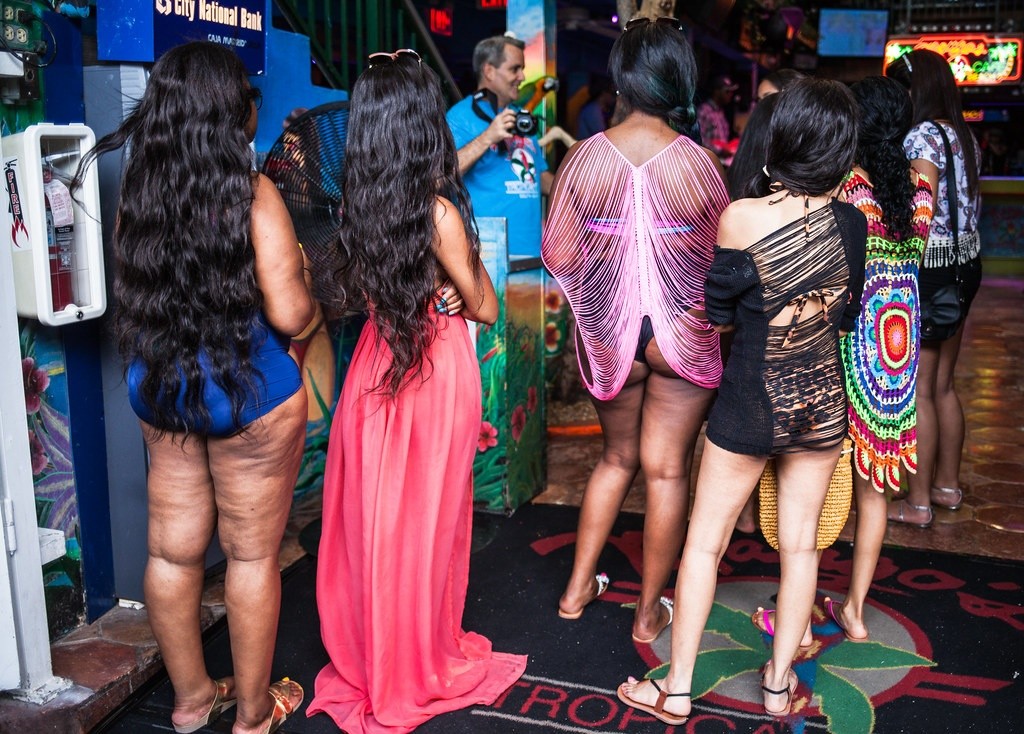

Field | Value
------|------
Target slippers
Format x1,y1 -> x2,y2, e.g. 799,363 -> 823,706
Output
824,597 -> 869,642
233,676 -> 303,734
752,607 -> 814,652
173,674 -> 239,734
632,594 -> 674,644
558,572 -> 609,619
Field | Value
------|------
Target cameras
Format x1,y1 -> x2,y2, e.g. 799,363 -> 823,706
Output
508,109 -> 539,138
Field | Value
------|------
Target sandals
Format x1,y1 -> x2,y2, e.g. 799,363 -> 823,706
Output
761,659 -> 798,717
616,676 -> 692,725
885,499 -> 936,529
929,483 -> 963,511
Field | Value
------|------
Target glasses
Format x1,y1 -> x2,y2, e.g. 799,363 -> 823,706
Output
624,16 -> 683,33
244,87 -> 263,110
369,49 -> 421,75
894,47 -> 912,73
719,86 -> 732,95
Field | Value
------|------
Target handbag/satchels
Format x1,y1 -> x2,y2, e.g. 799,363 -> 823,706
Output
920,285 -> 961,342
759,438 -> 853,553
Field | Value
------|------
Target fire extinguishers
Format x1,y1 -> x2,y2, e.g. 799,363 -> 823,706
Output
42,149 -> 82,312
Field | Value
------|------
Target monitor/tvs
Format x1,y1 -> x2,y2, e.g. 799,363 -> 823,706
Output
818,8 -> 890,57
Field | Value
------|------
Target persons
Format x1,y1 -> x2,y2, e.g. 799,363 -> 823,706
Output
445,37 -> 556,263
541,16 -> 727,642
71,41 -> 312,733
929,220 -> 936,223
306,48 -> 528,734
576,74 -> 614,141
886,49 -> 982,529
618,67 -> 934,724
980,123 -> 1024,177
